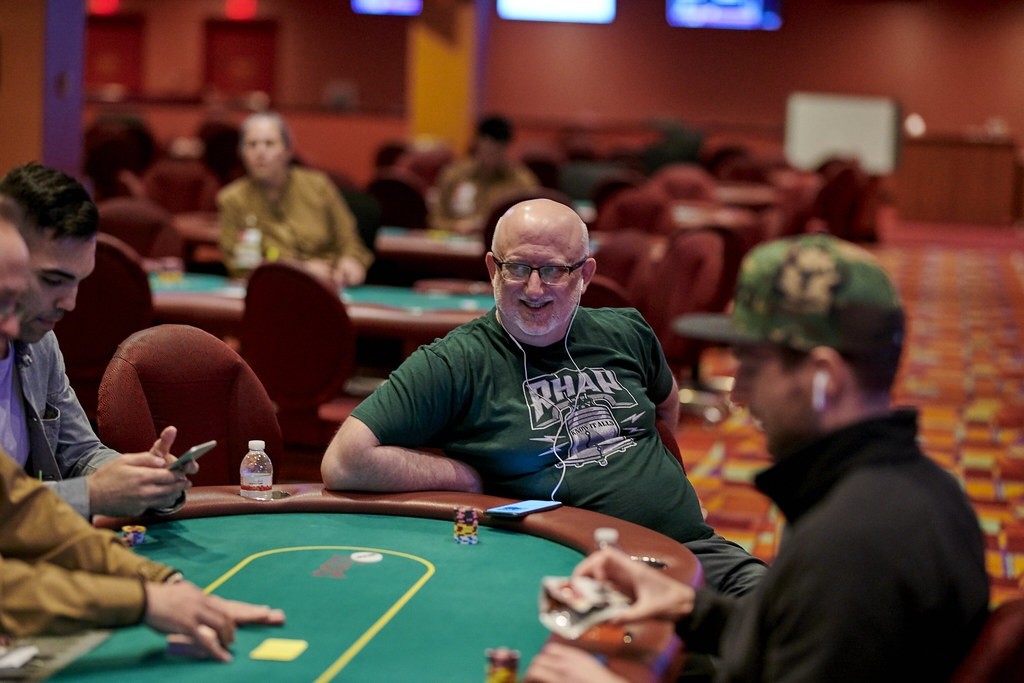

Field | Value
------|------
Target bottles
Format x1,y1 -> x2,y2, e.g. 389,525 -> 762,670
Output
239,440 -> 273,502
589,526 -> 621,556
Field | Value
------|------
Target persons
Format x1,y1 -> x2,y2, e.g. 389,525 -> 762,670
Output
525,234 -> 993,683
425,112 -> 537,231
214,115 -> 371,288
0,162 -> 198,526
321,198 -> 771,597
0,194 -> 287,664
80,82 -> 160,202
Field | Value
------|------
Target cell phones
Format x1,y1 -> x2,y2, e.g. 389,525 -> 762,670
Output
167,440 -> 217,471
482,499 -> 563,517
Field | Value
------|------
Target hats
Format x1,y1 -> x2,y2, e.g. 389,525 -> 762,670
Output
671,235 -> 904,361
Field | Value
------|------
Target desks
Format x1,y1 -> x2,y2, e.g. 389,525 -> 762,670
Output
0,483 -> 705,683
146,271 -> 497,379
374,228 -> 492,283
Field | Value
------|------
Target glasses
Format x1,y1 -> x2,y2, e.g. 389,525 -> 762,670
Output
491,255 -> 588,286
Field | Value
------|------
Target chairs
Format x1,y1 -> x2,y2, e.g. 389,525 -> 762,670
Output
53,143 -> 1024,683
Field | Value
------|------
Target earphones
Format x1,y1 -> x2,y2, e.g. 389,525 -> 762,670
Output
580,277 -> 584,291
813,371 -> 833,410
493,275 -> 497,290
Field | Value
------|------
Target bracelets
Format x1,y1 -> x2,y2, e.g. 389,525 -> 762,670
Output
167,573 -> 183,584
